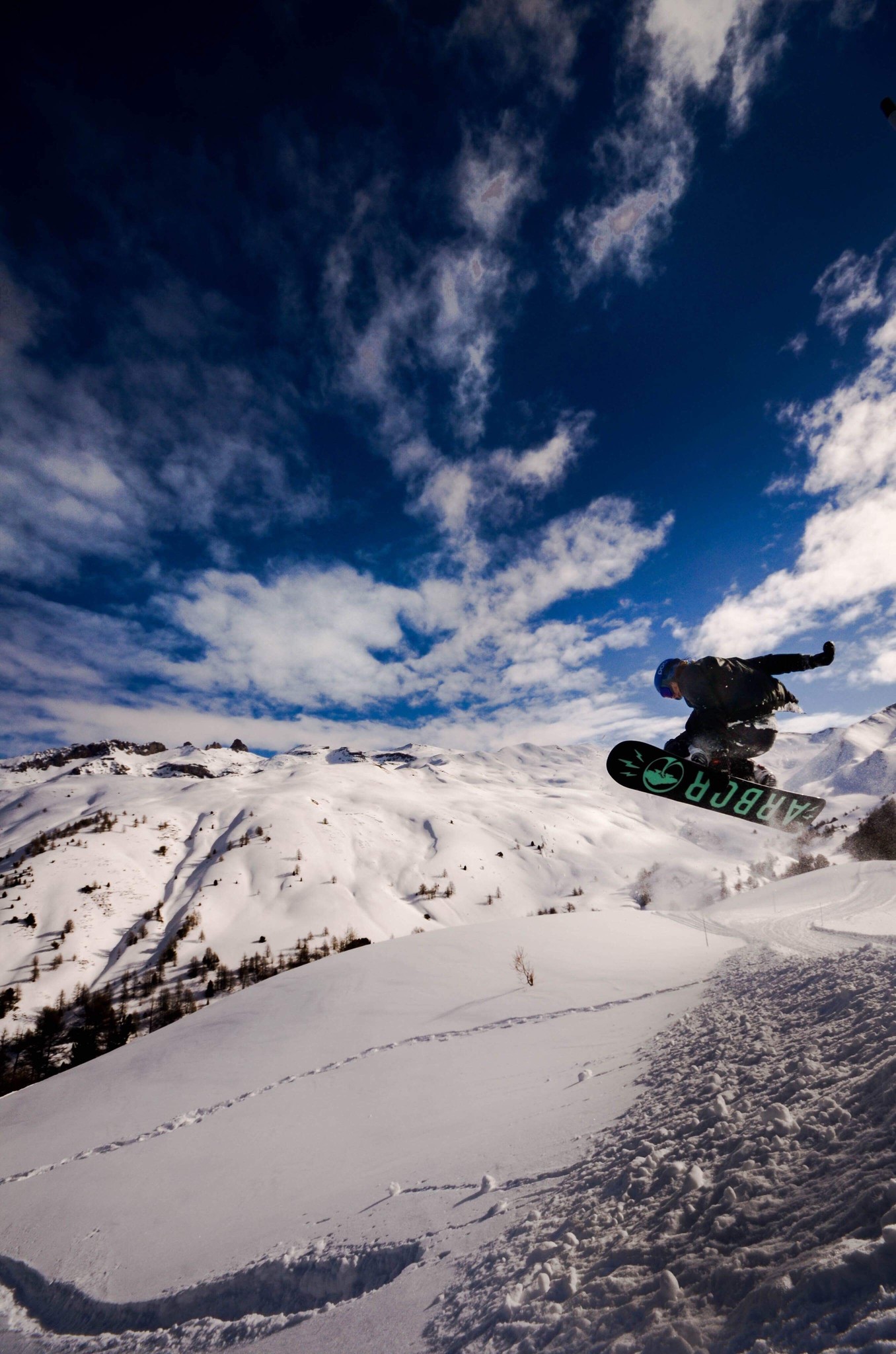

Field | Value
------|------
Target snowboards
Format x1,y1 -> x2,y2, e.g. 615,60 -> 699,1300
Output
603,740 -> 826,835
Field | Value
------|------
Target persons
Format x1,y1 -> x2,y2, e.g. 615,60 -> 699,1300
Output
654,641 -> 835,790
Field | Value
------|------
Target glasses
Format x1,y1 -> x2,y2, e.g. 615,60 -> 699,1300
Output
659,687 -> 672,698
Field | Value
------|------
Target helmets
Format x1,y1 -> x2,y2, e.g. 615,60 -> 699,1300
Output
654,658 -> 682,700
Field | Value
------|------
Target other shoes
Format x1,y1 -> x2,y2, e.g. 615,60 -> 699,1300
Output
752,762 -> 776,787
684,744 -> 708,767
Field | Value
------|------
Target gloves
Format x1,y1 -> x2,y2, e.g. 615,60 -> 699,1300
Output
802,641 -> 835,670
